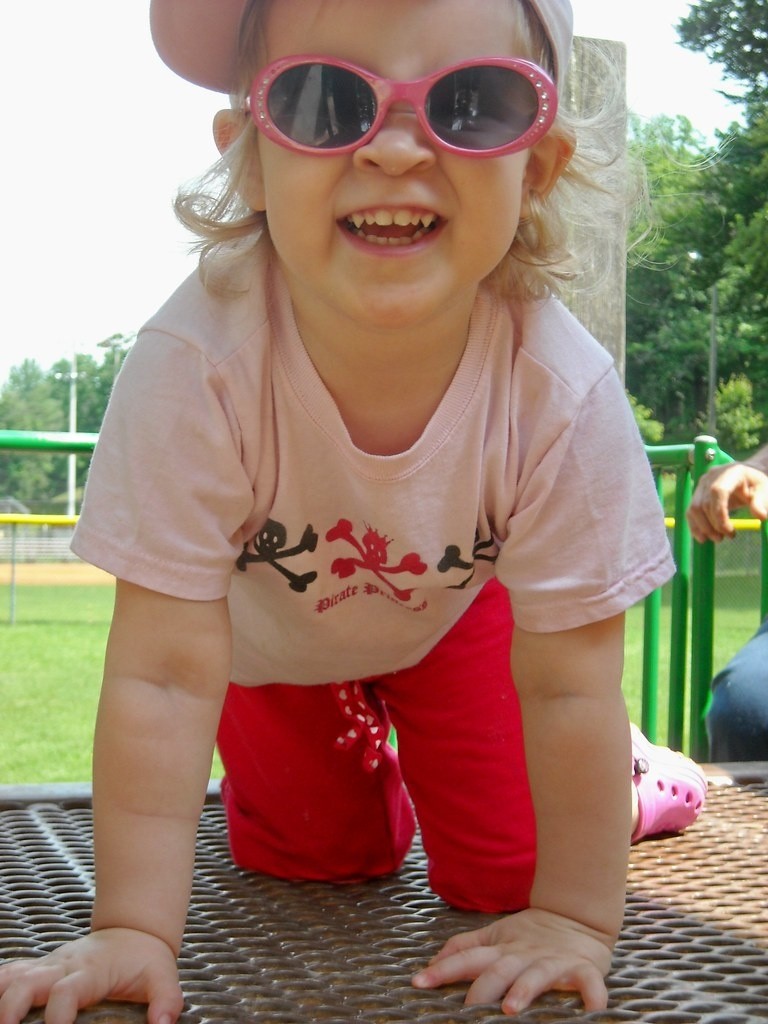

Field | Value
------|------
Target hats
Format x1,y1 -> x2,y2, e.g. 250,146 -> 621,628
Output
151,0 -> 574,87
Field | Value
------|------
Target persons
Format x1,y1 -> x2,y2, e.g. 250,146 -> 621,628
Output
0,0 -> 707,1023
687,443 -> 768,762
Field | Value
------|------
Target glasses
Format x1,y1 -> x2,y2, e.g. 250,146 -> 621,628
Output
243,54 -> 558,158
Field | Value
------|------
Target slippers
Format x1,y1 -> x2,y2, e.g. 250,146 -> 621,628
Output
630,723 -> 707,844
